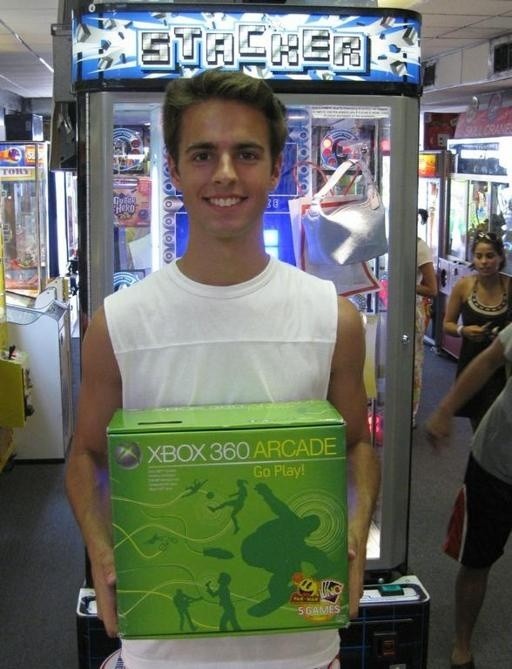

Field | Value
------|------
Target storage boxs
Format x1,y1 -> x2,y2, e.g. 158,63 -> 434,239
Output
106,400 -> 349,640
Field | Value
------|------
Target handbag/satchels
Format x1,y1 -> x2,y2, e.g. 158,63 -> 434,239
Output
288,185 -> 386,298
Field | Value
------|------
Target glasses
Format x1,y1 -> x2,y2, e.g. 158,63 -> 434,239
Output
476,231 -> 502,245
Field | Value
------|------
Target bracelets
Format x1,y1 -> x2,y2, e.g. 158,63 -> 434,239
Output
456,324 -> 464,339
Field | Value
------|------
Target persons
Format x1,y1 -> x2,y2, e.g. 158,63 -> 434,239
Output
440,233 -> 510,436
62,65 -> 381,669
423,319 -> 510,668
410,208 -> 439,429
